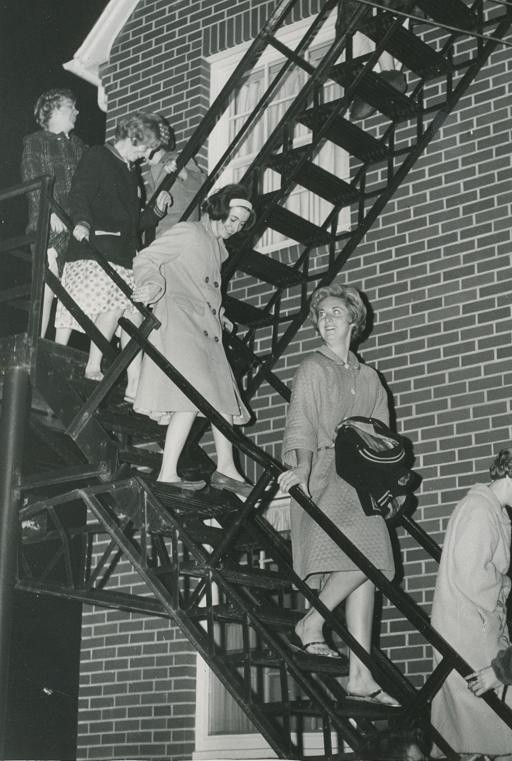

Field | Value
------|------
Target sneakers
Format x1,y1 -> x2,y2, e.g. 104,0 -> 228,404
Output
156,478 -> 207,491
209,470 -> 254,496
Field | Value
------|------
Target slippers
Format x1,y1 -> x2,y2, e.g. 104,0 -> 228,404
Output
344,687 -> 402,709
288,640 -> 341,660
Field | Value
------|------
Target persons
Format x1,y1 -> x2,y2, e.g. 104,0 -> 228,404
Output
264,283 -> 407,713
20,88 -> 90,340
129,183 -> 262,504
48,112 -> 172,406
423,445 -> 511,761
137,106 -> 210,251
461,645 -> 511,698
